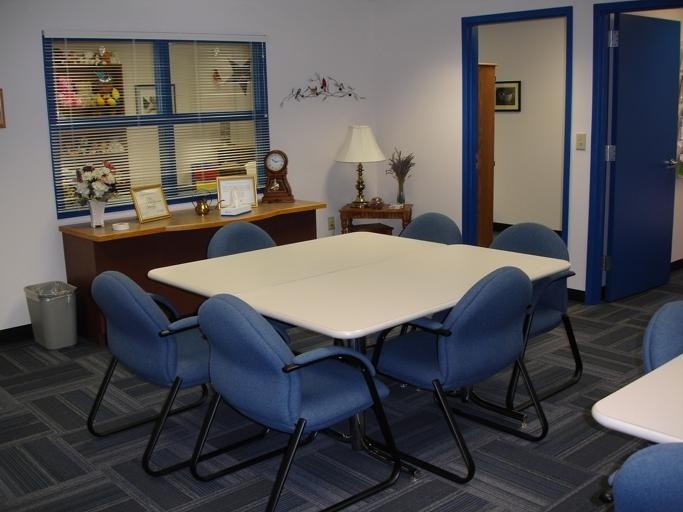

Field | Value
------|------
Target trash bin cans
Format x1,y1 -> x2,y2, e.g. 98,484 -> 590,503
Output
23,280 -> 78,351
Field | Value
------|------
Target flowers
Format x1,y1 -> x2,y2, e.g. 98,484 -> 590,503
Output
74,161 -> 121,206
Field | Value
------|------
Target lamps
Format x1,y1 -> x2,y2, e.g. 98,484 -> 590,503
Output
336,124 -> 386,208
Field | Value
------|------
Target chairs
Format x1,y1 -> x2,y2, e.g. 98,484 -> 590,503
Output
88,269 -> 272,478
611,443 -> 683,512
361,266 -> 551,486
467,221 -> 584,412
394,213 -> 464,244
643,301 -> 682,373
207,219 -> 278,260
188,292 -> 404,511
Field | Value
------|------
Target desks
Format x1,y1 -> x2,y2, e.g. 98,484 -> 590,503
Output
147,228 -> 573,347
339,203 -> 413,234
59,198 -> 328,347
591,353 -> 683,503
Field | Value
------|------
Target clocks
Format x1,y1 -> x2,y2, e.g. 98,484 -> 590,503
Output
260,150 -> 294,202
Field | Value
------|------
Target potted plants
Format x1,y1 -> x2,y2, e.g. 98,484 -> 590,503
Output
384,146 -> 416,204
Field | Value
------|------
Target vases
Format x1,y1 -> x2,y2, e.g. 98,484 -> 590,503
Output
87,199 -> 106,229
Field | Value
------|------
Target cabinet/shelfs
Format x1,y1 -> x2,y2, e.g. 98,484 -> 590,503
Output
478,62 -> 496,247
51,65 -> 131,196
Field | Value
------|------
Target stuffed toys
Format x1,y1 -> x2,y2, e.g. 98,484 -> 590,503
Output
52,44 -> 129,202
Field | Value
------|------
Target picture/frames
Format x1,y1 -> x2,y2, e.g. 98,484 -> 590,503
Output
129,184 -> 172,224
216,174 -> 258,210
134,84 -> 176,115
495,80 -> 521,113
